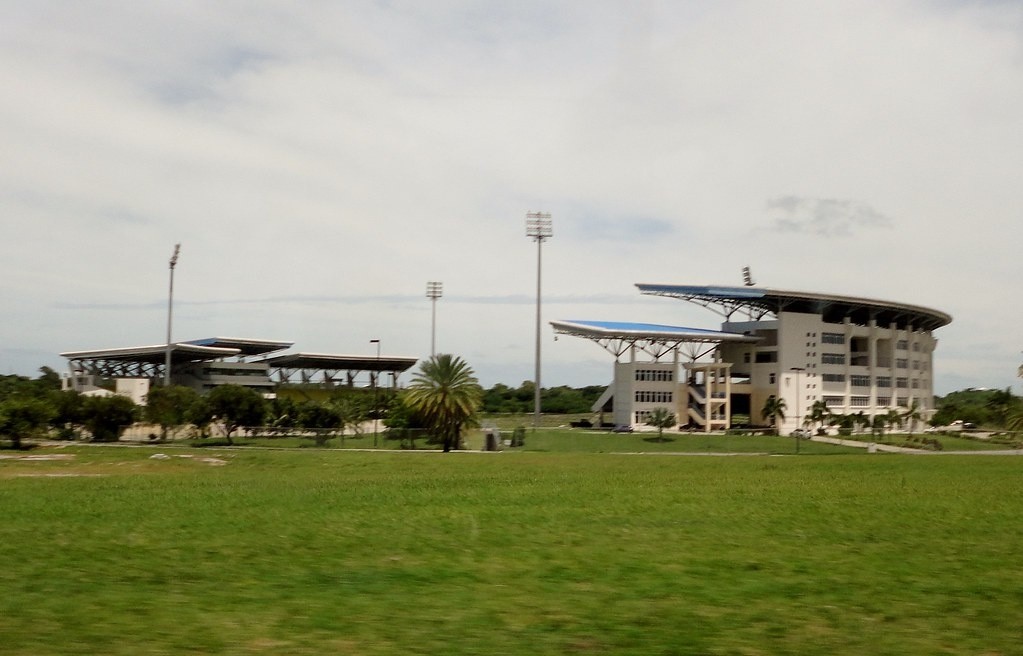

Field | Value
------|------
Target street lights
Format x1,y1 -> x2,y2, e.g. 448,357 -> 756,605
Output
524,208 -> 554,413
789,366 -> 805,454
162,242 -> 184,386
369,338 -> 381,447
426,280 -> 444,358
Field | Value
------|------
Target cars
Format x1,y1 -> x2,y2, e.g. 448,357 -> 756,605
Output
789,429 -> 813,439
